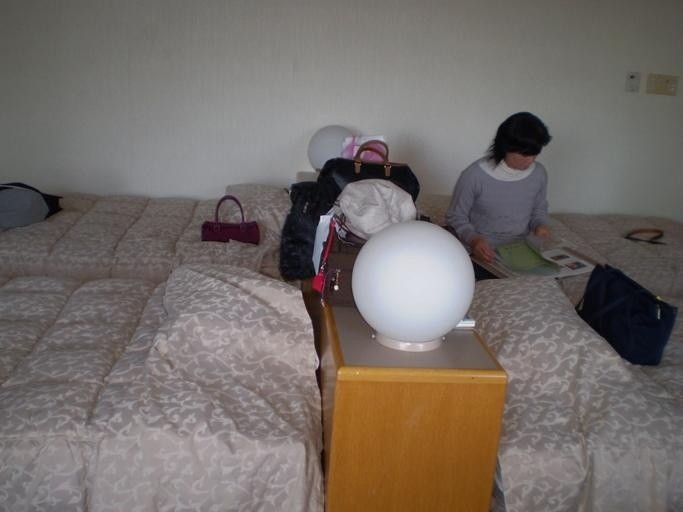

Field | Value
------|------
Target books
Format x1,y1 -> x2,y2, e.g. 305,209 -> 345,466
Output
469,238 -> 599,280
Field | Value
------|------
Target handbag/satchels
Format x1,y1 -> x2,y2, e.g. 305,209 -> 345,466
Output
280,158 -> 421,306
574,262 -> 677,367
202,221 -> 260,244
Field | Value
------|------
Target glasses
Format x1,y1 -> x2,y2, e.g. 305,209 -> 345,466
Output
626,229 -> 663,244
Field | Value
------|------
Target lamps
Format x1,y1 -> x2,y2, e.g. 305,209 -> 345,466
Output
307,124 -> 353,172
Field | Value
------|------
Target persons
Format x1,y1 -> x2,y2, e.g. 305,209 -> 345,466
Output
446,111 -> 553,280
